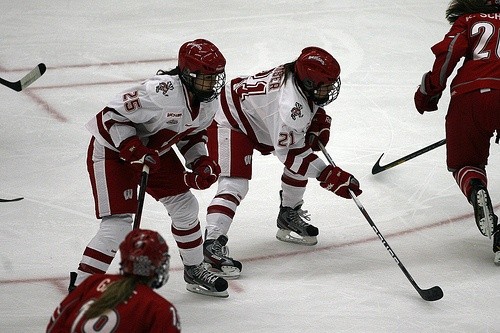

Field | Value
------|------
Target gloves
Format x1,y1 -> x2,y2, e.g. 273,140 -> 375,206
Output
119,138 -> 159,172
181,155 -> 221,190
305,108 -> 332,151
316,165 -> 362,199
414,70 -> 446,115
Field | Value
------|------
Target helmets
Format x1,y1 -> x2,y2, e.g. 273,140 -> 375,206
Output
295,46 -> 341,107
178,38 -> 226,103
119,229 -> 171,289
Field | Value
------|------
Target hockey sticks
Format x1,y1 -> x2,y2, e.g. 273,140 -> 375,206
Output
0,197 -> 25,204
0,63 -> 46,93
371,136 -> 450,176
321,143 -> 447,303
131,161 -> 147,230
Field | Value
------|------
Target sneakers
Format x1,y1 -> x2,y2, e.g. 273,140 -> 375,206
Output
493,223 -> 500,262
469,181 -> 496,240
184,264 -> 229,297
275,190 -> 319,246
203,229 -> 242,279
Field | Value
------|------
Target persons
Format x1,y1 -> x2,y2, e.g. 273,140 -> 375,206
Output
68,37 -> 230,298
414,0 -> 500,265
45,229 -> 181,333
203,47 -> 362,280
